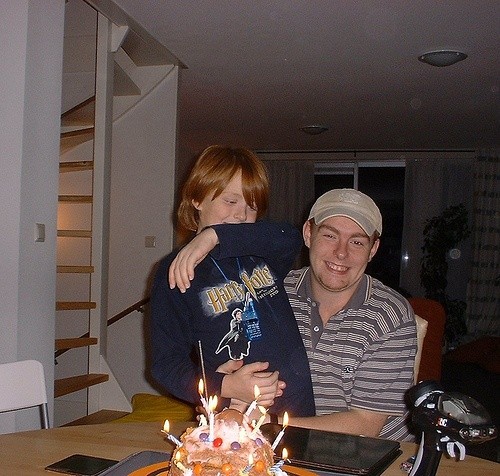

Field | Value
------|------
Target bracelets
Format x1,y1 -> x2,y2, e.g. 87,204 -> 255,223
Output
268,413 -> 279,424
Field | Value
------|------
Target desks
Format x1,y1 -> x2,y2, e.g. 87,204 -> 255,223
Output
0,421 -> 500,476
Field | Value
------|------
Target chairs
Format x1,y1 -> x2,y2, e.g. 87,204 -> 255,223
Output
0,359 -> 50,429
401,298 -> 445,392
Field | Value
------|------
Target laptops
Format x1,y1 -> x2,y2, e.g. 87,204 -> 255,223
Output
259,423 -> 403,476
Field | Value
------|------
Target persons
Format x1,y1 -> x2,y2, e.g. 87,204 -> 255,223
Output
193,189 -> 418,439
143,146 -> 316,421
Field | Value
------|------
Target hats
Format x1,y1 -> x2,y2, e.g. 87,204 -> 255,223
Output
307,188 -> 383,237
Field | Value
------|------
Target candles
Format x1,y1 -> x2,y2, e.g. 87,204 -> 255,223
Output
272,411 -> 289,451
270,448 -> 291,472
246,384 -> 260,418
198,379 -> 208,413
254,409 -> 269,434
208,396 -> 215,437
160,419 -> 182,446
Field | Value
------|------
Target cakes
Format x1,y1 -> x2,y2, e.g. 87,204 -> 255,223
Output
168,423 -> 276,476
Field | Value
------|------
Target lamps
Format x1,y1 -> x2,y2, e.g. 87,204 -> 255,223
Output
417,50 -> 468,67
301,126 -> 328,135
